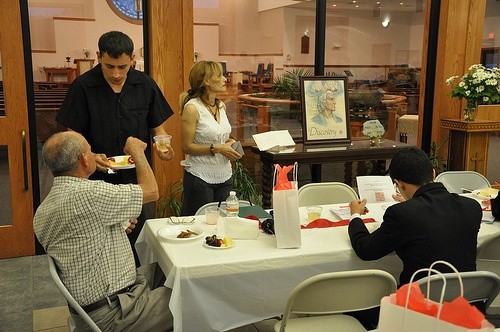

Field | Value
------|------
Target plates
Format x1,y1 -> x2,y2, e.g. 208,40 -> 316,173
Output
108,155 -> 136,169
202,238 -> 236,248
157,225 -> 204,241
471,188 -> 499,200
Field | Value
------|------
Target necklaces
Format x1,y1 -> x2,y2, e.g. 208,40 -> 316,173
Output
199,95 -> 219,121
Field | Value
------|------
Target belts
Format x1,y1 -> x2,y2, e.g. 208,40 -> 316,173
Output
68,284 -> 135,315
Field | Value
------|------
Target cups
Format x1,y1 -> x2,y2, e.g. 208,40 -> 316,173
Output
369,134 -> 381,147
306,205 -> 322,221
153,135 -> 172,152
205,207 -> 220,224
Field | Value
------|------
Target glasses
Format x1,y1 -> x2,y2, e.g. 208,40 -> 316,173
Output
396,185 -> 400,193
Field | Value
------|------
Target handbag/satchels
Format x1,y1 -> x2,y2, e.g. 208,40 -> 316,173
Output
377,261 -> 494,332
273,162 -> 301,248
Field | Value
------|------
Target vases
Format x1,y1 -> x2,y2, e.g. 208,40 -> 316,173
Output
462,98 -> 479,122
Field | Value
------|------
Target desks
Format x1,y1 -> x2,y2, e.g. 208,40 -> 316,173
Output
42,66 -> 78,82
134,191 -> 500,332
251,140 -> 414,210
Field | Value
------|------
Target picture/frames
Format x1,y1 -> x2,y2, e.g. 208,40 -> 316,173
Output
299,76 -> 352,145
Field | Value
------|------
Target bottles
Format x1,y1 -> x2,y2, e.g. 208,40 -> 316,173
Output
226,191 -> 240,217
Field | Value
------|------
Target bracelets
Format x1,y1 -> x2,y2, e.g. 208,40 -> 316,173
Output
210,144 -> 215,157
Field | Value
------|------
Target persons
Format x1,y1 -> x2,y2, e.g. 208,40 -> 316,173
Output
348,147 -> 482,289
54,31 -> 176,269
32,131 -> 174,332
179,61 -> 242,217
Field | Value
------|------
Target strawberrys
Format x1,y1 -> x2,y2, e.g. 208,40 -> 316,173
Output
129,157 -> 136,164
217,239 -> 223,246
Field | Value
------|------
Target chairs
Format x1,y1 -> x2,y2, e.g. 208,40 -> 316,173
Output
47,254 -> 102,332
298,181 -> 359,206
238,63 -> 274,95
435,171 -> 491,192
273,268 -> 500,332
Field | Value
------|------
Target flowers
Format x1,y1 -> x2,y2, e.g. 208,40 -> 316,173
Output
445,63 -> 500,111
